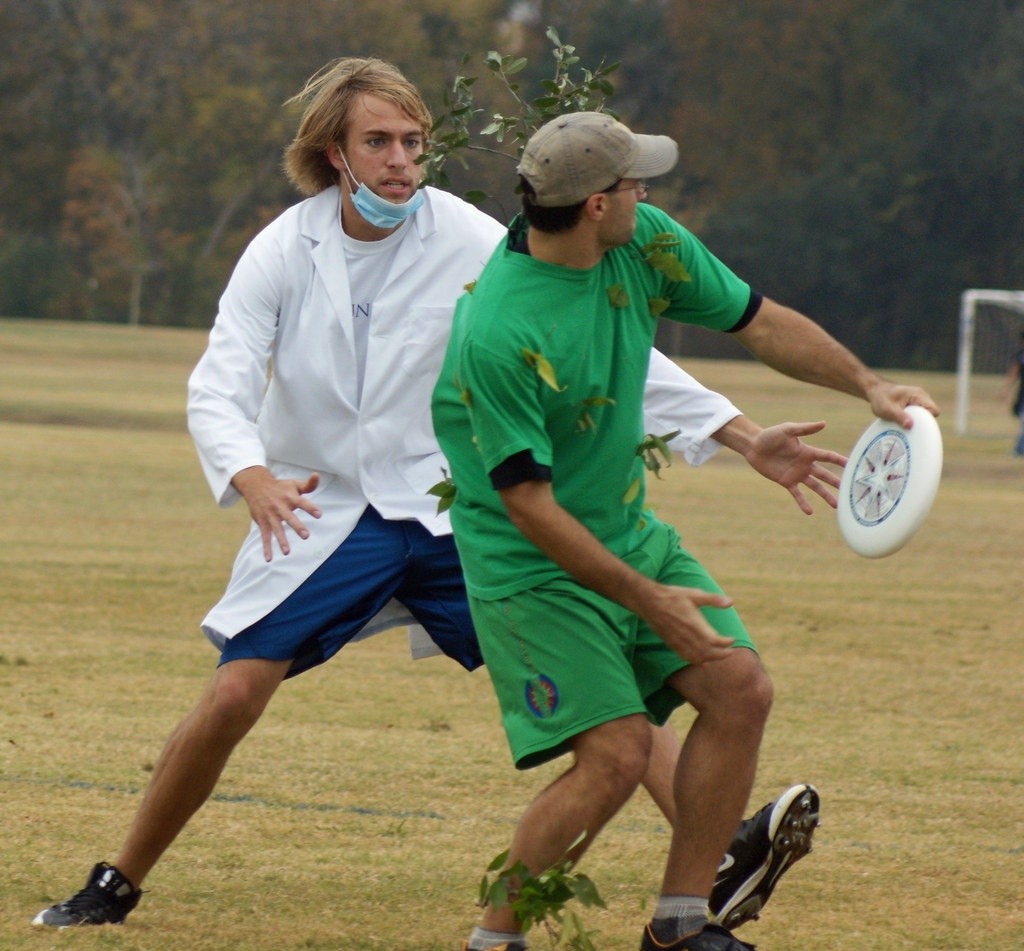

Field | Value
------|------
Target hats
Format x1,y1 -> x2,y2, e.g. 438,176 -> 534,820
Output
512,111 -> 680,207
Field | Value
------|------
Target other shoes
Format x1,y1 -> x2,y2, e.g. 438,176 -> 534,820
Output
639,921 -> 757,951
458,940 -> 529,951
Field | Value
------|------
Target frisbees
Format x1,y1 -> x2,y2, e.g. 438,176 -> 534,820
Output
833,405 -> 948,561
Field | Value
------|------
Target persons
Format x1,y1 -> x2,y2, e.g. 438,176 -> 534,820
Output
431,111 -> 941,951
1001,350 -> 1024,456
34,59 -> 850,932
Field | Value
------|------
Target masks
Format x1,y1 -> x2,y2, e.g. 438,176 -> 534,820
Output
337,144 -> 425,229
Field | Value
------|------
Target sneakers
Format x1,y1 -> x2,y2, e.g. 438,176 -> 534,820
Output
707,783 -> 821,932
31,861 -> 152,928
